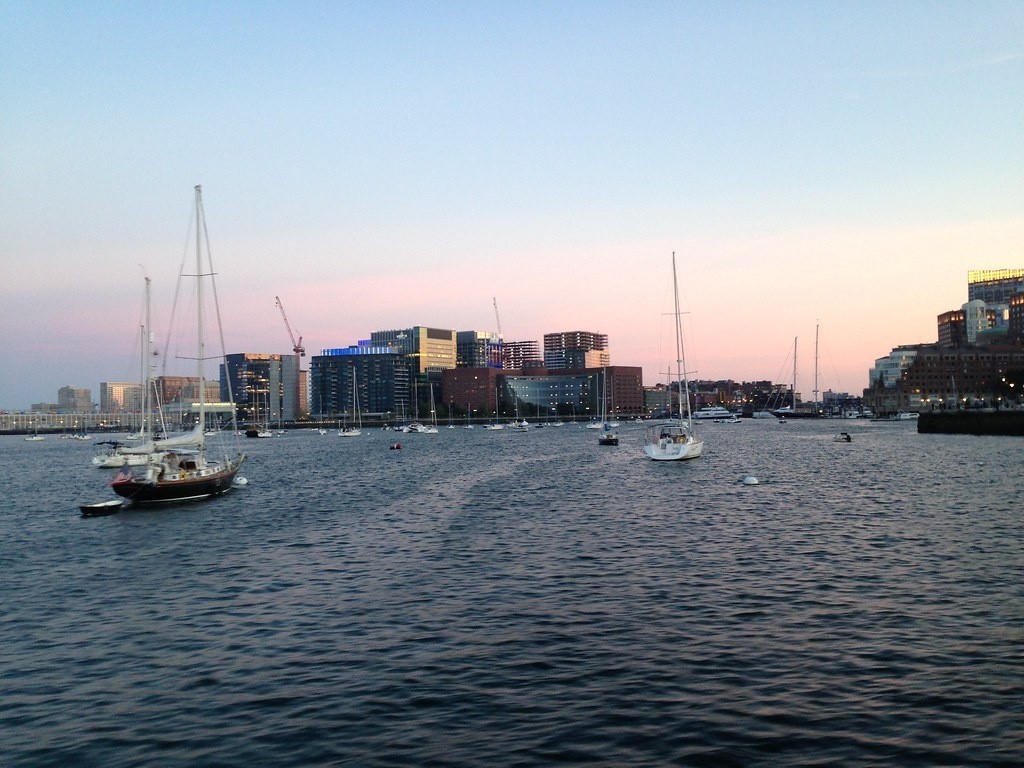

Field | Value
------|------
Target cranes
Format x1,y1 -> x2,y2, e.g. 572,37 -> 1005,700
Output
275,297 -> 305,356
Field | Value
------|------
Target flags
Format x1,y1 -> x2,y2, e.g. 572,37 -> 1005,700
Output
105,462 -> 135,488
653,434 -> 659,443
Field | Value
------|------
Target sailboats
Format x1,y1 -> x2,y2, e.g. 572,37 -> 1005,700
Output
244,384 -> 274,438
642,251 -> 705,460
92,277 -> 206,466
339,365 -> 362,437
463,403 -> 473,428
598,365 -> 620,445
487,386 -> 622,430
382,377 -> 438,435
444,403 -> 455,429
109,184 -> 246,504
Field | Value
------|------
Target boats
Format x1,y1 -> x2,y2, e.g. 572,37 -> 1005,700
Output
70,420 -> 93,440
901,413 -> 917,420
693,402 -> 742,417
26,435 -> 44,442
